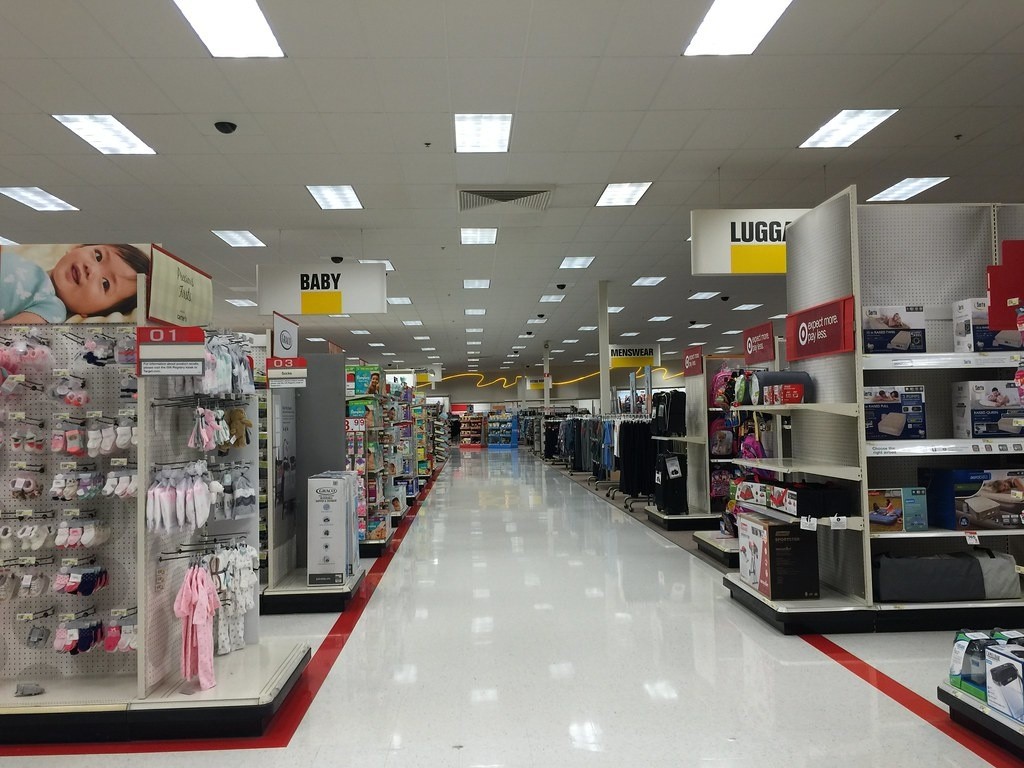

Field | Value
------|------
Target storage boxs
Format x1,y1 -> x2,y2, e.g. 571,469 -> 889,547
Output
766,482 -> 828,519
949,629 -> 1000,702
949,380 -> 1024,438
951,298 -> 1024,352
984,644 -> 1024,726
736,512 -> 821,601
324,470 -> 360,576
726,478 -> 778,508
308,475 -> 350,586
863,305 -> 927,356
863,385 -> 926,441
905,488 -> 928,532
869,487 -> 904,533
990,628 -> 1024,646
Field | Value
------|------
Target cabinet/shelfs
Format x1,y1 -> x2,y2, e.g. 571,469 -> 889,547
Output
458,414 -> 487,447
642,183 -> 1024,763
0,181 -> 453,768
487,411 -> 519,448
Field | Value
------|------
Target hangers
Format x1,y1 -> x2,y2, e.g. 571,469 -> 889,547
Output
563,413 -> 651,426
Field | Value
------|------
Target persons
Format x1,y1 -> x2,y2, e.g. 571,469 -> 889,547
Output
389,463 -> 396,474
392,497 -> 401,511
0,244 -> 149,323
871,390 -> 898,402
988,387 -> 1009,406
366,373 -> 379,394
887,313 -> 901,325
375,528 -> 386,539
389,407 -> 395,420
